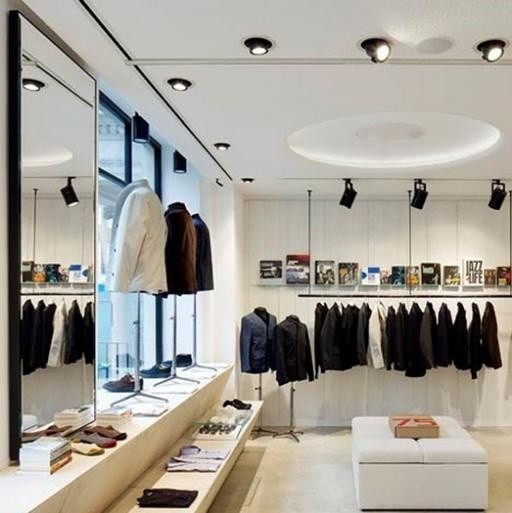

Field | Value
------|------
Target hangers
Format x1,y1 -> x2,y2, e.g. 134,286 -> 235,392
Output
318,295 -> 382,306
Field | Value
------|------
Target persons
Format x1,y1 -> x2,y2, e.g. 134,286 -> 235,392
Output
164,202 -> 198,296
273,314 -> 314,387
105,177 -> 168,296
192,213 -> 215,292
240,306 -> 277,375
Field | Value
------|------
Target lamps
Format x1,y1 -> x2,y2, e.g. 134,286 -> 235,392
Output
132,112 -> 149,144
60,176 -> 80,208
22,80 -> 40,91
339,178 -> 506,210
361,39 -> 390,62
478,40 -> 504,63
173,150 -> 186,173
171,80 -> 188,92
249,41 -> 268,56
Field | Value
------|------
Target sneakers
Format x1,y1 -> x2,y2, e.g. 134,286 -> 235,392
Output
102,354 -> 192,393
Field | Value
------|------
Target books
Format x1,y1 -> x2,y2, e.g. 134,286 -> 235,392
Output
192,424 -> 243,440
96,406 -> 133,426
193,408 -> 254,427
52,406 -> 92,428
15,436 -> 73,476
388,414 -> 441,439
21,415 -> 39,433
258,252 -> 511,288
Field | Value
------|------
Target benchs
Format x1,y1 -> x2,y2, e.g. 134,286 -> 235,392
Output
349,415 -> 490,511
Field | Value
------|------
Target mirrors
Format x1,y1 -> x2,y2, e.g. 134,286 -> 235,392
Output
8,9 -> 99,469
